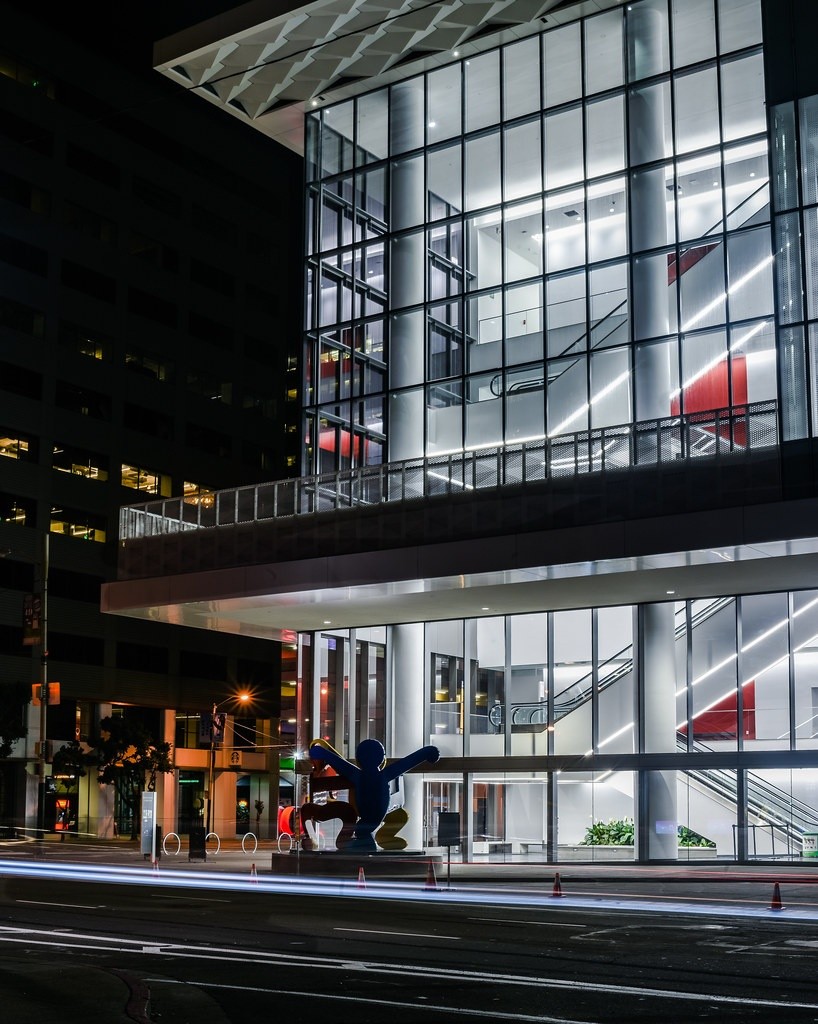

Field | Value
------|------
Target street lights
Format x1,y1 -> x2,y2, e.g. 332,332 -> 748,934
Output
206,689 -> 251,842
